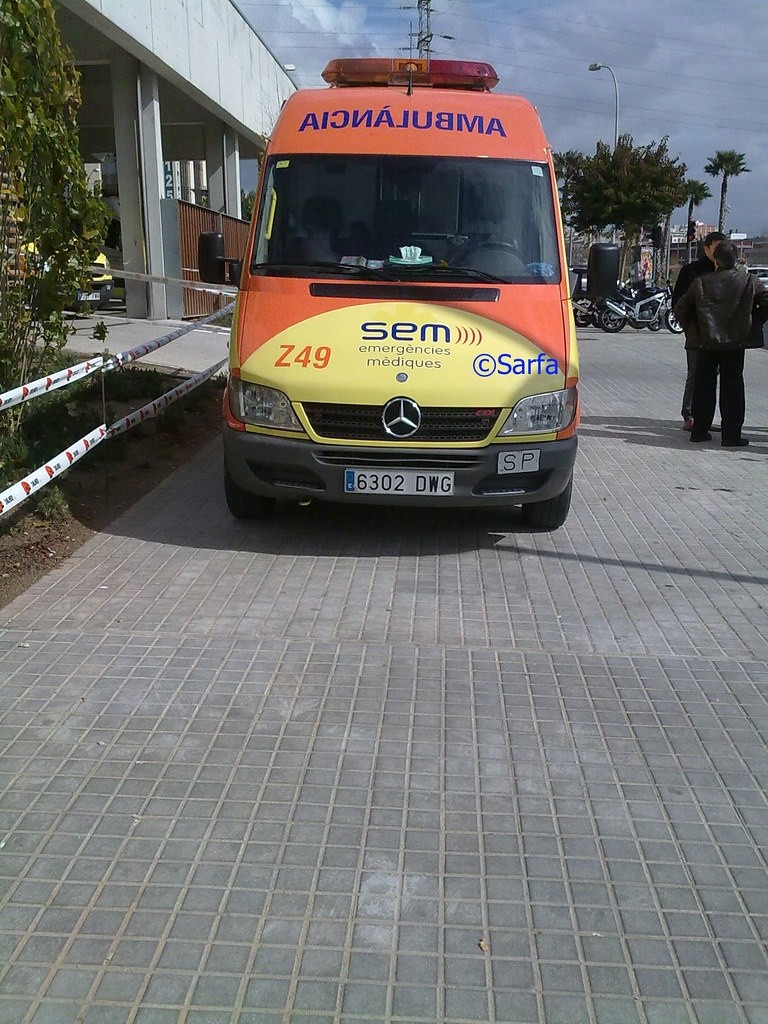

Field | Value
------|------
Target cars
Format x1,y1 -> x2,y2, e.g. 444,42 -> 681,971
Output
746,267 -> 768,290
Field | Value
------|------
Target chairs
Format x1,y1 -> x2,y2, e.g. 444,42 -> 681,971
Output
283,185 -> 375,267
420,163 -> 525,267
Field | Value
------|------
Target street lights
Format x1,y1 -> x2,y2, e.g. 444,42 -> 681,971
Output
589,62 -> 621,161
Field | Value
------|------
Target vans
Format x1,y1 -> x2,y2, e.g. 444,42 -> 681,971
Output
198,48 -> 584,530
11,183 -> 115,317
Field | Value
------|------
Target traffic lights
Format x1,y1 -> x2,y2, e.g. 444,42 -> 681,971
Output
687,221 -> 696,242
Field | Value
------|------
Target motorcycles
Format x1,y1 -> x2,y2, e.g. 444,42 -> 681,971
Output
569,241 -> 685,335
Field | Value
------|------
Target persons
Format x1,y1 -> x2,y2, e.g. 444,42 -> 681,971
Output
739,258 -> 748,274
671,232 -> 726,432
674,240 -> 768,448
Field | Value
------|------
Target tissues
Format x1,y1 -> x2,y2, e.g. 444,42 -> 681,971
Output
387,245 -> 434,265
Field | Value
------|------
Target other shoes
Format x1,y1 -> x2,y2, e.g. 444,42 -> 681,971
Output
691,433 -> 712,442
685,419 -> 692,431
709,424 -> 722,432
721,438 -> 749,446
